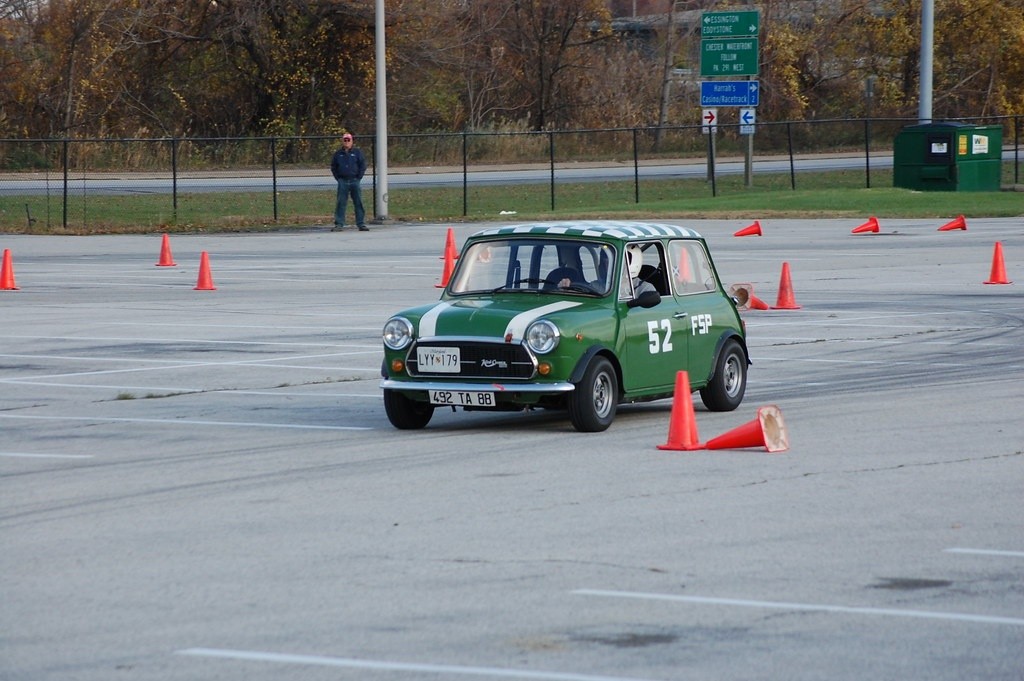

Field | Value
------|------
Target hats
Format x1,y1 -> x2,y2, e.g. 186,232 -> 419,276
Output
343,134 -> 352,140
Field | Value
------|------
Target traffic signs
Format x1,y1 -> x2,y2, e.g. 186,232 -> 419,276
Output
702,110 -> 718,135
739,109 -> 756,135
701,10 -> 759,39
699,81 -> 759,107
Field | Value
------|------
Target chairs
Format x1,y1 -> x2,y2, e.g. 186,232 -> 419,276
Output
542,267 -> 582,292
638,264 -> 666,296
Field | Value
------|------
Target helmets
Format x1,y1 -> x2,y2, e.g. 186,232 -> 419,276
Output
598,245 -> 643,280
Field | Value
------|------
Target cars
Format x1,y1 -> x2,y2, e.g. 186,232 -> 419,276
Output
378,219 -> 753,432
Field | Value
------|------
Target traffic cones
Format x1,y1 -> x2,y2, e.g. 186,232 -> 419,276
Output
0,249 -> 21,290
726,283 -> 769,311
434,228 -> 490,288
677,247 -> 692,282
983,242 -> 1013,285
733,220 -> 763,237
706,405 -> 789,452
656,371 -> 706,451
851,216 -> 881,233
192,250 -> 216,290
154,233 -> 177,266
769,262 -> 801,309
937,215 -> 968,232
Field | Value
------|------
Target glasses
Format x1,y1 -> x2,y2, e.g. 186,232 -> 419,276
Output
344,140 -> 351,142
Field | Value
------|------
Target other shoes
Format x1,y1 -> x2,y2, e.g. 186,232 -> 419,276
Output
331,226 -> 344,232
359,226 -> 369,231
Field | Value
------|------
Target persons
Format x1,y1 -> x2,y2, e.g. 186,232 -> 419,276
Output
556,243 -> 657,299
329,133 -> 368,232
